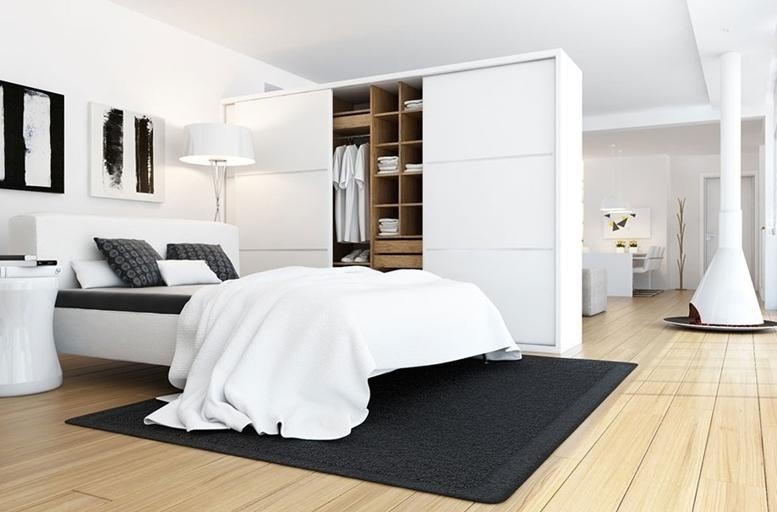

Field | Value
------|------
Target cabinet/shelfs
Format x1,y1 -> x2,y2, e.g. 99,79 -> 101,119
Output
221,48 -> 585,358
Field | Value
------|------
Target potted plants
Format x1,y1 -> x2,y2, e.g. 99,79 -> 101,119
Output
616,240 -> 625,254
630,240 -> 637,253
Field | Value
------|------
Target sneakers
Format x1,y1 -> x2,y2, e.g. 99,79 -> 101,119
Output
340,249 -> 369,263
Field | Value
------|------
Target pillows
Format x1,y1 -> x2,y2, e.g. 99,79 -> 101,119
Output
93,237 -> 165,285
71,258 -> 128,288
157,257 -> 223,286
165,243 -> 239,279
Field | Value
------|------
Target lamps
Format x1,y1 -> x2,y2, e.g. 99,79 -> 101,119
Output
178,121 -> 258,223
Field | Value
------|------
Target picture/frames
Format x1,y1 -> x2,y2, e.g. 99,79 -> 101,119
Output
2,81 -> 65,195
90,100 -> 166,202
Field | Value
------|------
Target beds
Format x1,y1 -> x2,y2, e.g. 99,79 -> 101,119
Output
13,209 -> 523,441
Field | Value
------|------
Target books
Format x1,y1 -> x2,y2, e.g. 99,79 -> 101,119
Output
0,258 -> 59,268
0,255 -> 37,260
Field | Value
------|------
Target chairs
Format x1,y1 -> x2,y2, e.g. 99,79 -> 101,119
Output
633,245 -> 666,297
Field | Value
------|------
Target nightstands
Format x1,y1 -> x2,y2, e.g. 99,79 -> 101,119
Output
0,267 -> 65,397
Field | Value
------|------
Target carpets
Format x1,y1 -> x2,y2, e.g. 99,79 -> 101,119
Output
63,354 -> 639,504
